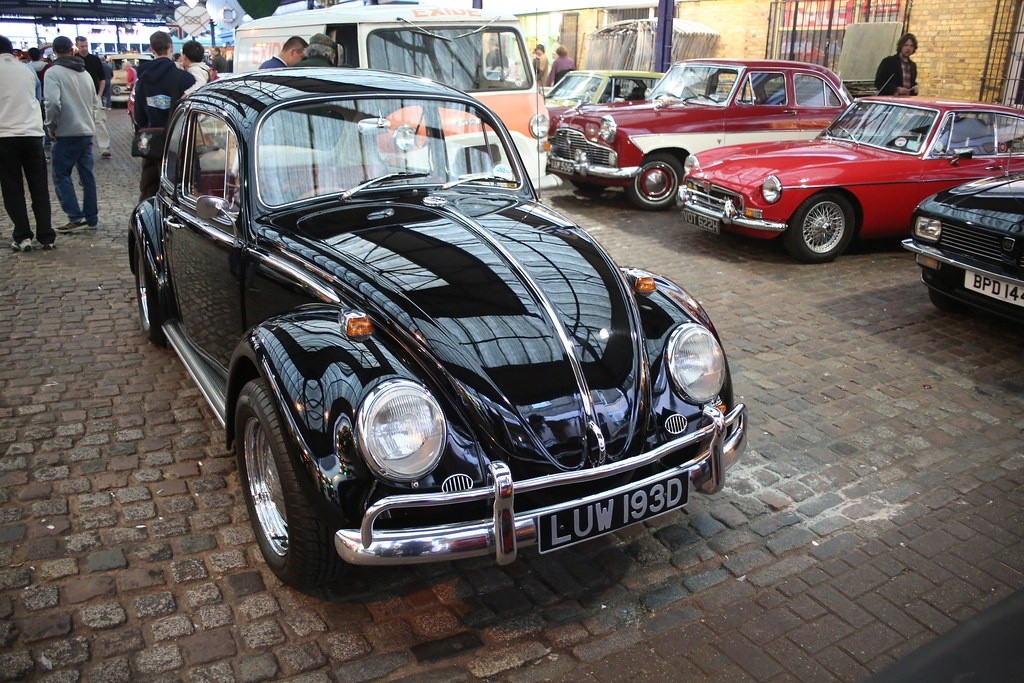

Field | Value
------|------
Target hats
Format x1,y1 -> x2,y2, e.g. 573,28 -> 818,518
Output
537,44 -> 544,52
310,32 -> 337,50
53,36 -> 74,52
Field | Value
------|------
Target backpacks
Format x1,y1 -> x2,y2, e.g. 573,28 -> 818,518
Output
186,64 -> 219,83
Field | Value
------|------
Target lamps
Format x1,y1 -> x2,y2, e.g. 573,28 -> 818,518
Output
134,20 -> 144,27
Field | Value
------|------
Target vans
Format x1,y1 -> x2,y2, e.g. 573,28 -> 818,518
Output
231,1 -> 564,193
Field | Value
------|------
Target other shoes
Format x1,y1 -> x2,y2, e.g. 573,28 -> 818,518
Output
101,148 -> 111,158
11,238 -> 33,252
38,241 -> 54,249
102,106 -> 111,110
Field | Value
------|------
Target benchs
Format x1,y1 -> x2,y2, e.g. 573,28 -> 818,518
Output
203,164 -> 344,199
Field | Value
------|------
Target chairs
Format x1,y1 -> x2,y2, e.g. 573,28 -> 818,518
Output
611,84 -> 645,101
903,114 -> 988,154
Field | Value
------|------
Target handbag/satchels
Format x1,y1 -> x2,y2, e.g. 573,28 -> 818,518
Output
130,128 -> 164,159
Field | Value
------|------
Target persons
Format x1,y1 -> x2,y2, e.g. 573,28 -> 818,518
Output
258,33 -> 339,72
12,36 -> 113,158
873,33 -> 920,97
0,34 -> 57,253
43,36 -> 99,233
122,32 -> 228,202
484,38 -> 510,82
532,44 -> 575,87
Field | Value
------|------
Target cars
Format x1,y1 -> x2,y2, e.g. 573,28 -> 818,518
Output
546,58 -> 855,210
675,95 -> 1024,263
105,56 -> 136,103
542,70 -> 665,108
126,67 -> 749,589
902,169 -> 1024,320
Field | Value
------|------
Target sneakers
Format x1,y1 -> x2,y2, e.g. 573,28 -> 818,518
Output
54,218 -> 97,232
